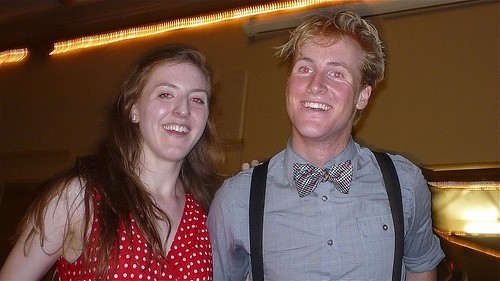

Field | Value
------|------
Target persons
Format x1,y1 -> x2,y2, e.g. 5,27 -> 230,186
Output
202,6 -> 445,281
1,41 -> 269,280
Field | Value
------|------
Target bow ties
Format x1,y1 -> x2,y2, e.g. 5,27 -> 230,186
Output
292,159 -> 352,198
